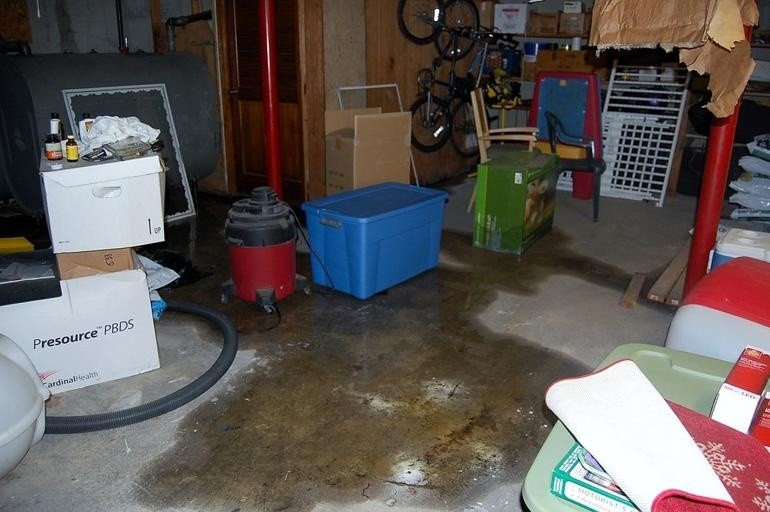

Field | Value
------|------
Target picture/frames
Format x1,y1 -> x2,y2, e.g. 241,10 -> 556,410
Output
61,83 -> 198,224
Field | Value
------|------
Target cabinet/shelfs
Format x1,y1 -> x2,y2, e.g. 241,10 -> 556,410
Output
486,100 -> 530,127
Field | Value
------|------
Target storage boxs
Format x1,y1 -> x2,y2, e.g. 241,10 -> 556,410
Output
559,13 -> 591,33
563,2 -> 588,14
529,10 -> 564,34
325,107 -> 412,196
39,139 -> 167,254
0,250 -> 160,395
493,4 -> 538,34
473,151 -> 572,255
523,42 -> 608,82
301,182 -> 449,299
55,248 -> 135,280
521,344 -> 770,511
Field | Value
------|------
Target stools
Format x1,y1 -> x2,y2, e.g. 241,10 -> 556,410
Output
664,258 -> 770,358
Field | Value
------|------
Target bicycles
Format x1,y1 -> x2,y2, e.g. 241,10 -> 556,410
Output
397,0 -> 527,160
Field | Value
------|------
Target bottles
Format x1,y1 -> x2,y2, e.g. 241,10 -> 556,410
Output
45,113 -> 79,163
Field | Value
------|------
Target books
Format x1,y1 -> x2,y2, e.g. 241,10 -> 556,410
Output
550,442 -> 642,512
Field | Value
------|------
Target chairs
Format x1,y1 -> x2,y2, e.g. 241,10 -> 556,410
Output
466,89 -> 540,215
526,71 -> 606,222
